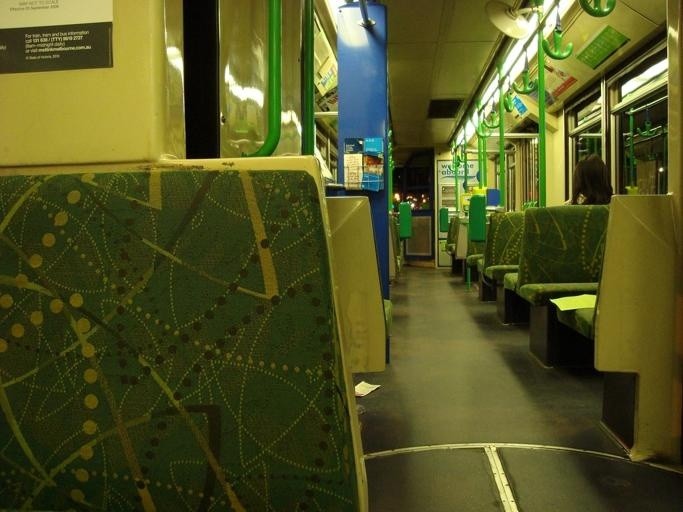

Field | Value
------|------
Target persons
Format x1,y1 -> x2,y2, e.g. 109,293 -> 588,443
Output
559,154 -> 615,205
